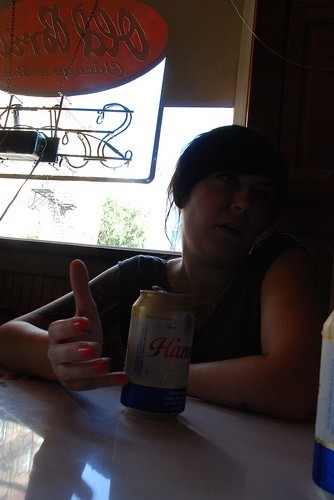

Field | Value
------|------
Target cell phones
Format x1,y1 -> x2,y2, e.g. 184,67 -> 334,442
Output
247,219 -> 275,259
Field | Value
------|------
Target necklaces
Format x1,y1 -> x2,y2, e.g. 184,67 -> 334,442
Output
194,280 -> 235,318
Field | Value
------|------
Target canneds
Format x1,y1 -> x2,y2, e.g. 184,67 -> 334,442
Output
120,287 -> 200,422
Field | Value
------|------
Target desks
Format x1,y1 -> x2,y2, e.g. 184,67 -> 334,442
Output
0,372 -> 334,500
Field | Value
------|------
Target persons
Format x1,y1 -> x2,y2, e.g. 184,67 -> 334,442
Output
1,377 -> 314,500
0,125 -> 320,419
